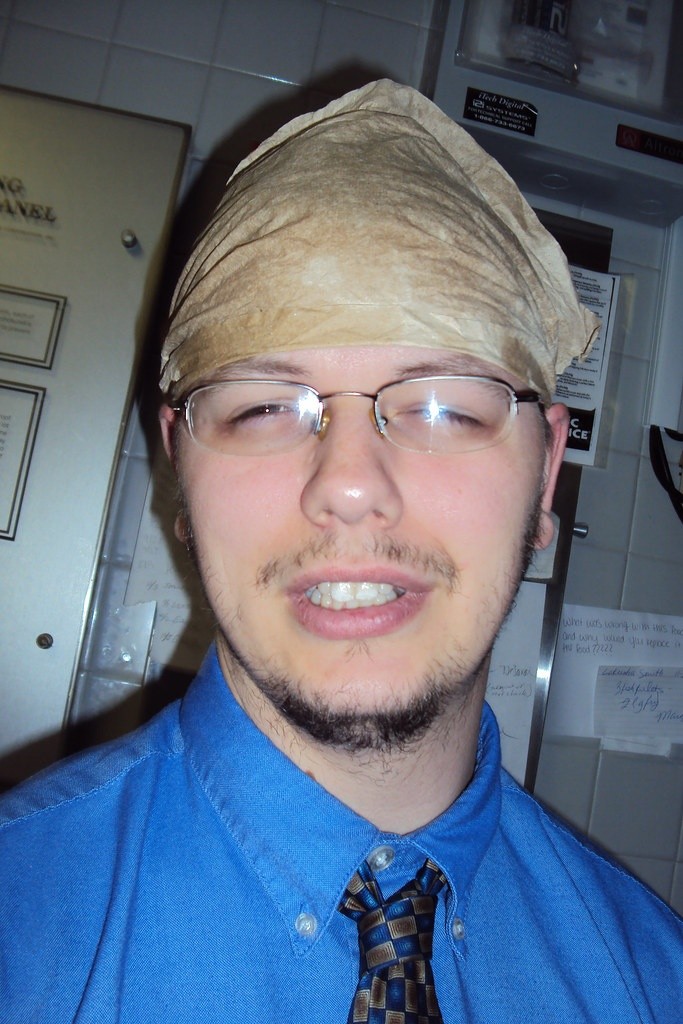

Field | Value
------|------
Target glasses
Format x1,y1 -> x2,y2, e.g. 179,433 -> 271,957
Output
165,371 -> 538,458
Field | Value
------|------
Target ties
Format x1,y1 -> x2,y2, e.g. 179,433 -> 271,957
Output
338,858 -> 446,1024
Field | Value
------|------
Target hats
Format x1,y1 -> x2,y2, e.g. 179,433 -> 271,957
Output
159,77 -> 603,408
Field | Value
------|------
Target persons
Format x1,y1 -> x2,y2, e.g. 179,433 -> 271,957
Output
0,109 -> 683,1024
